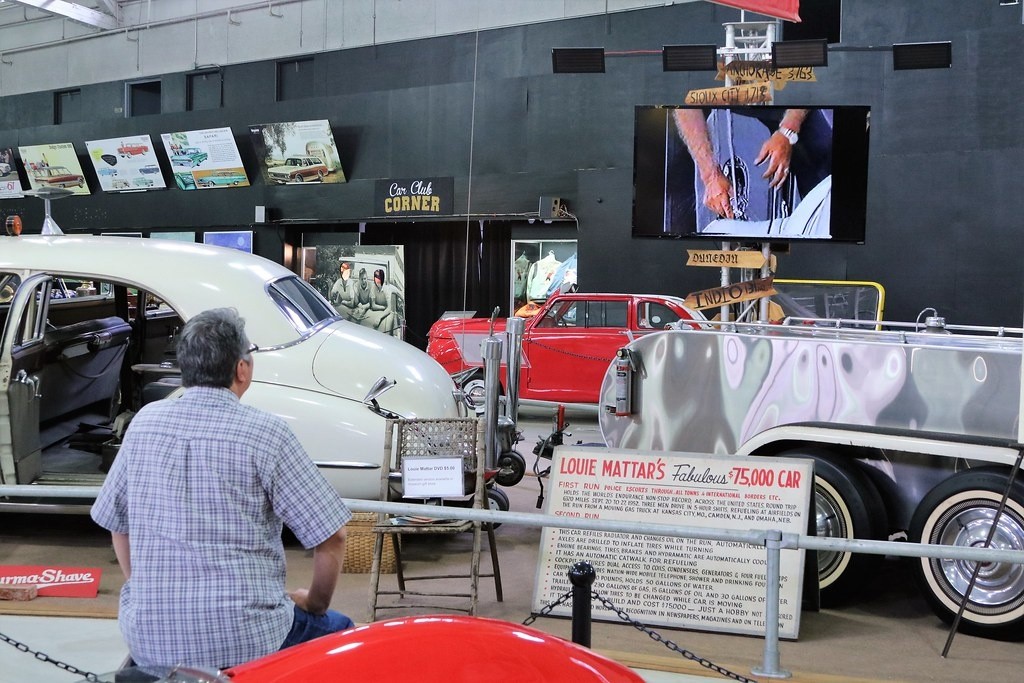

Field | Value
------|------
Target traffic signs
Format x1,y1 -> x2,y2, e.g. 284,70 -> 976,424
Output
686,247 -> 766,269
683,80 -> 773,106
680,275 -> 780,312
721,60 -> 816,84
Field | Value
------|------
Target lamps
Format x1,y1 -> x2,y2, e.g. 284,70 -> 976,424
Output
773,38 -> 829,68
551,47 -> 607,74
892,40 -> 953,70
662,44 -> 717,72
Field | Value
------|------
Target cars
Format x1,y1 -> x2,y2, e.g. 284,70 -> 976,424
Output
112,178 -> 131,188
118,143 -> 149,158
196,170 -> 246,188
63,612 -> 645,683
0,155 -> 12,177
170,147 -> 208,168
97,166 -> 119,177
34,166 -> 86,190
132,177 -> 154,187
0,217 -> 526,536
139,164 -> 159,175
267,153 -> 329,185
424,289 -> 721,417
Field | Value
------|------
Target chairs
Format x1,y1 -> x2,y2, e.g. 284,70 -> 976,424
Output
37,315 -> 134,449
366,415 -> 504,625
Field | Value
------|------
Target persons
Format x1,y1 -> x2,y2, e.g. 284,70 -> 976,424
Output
88,308 -> 353,674
329,263 -> 396,337
672,108 -> 809,232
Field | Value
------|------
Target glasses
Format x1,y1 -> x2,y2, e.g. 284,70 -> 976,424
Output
246,343 -> 259,355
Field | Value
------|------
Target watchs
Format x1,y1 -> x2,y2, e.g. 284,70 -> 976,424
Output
778,126 -> 800,144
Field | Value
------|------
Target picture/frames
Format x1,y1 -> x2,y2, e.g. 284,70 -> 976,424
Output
510,238 -> 577,328
301,245 -> 318,292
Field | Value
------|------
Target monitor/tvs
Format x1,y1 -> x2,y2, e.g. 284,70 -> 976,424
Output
629,104 -> 871,245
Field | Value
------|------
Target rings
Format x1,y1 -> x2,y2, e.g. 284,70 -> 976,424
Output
784,168 -> 790,173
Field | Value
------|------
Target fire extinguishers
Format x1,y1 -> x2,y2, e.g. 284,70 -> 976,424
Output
615,347 -> 637,416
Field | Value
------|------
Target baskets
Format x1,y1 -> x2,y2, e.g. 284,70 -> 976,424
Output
342,511 -> 402,575
